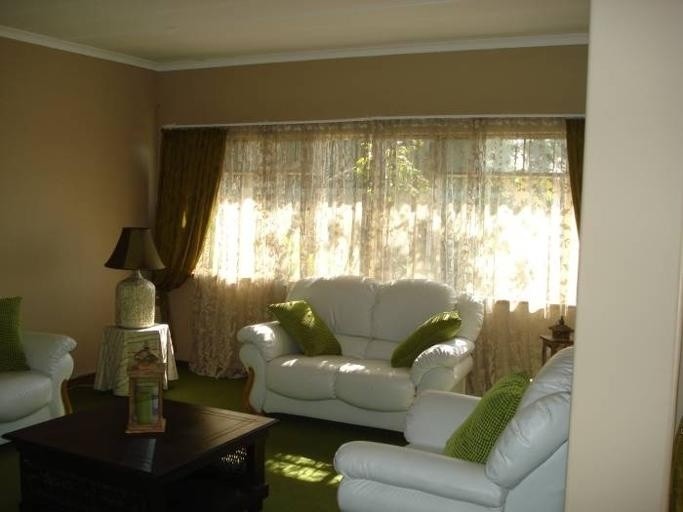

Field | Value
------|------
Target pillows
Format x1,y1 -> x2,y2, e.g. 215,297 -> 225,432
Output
391,310 -> 464,369
266,296 -> 342,357
0,296 -> 31,372
442,371 -> 530,465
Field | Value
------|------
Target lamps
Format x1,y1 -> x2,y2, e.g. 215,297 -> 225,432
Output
103,226 -> 166,330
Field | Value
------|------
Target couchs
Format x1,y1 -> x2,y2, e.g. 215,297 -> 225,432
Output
236,273 -> 484,433
333,345 -> 575,512
0,331 -> 78,447
